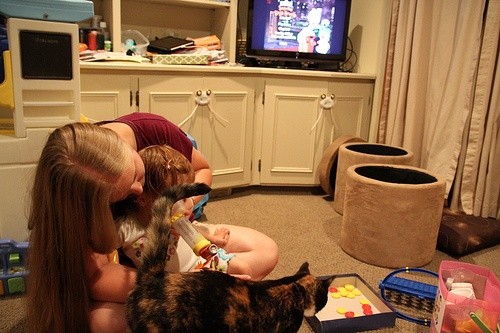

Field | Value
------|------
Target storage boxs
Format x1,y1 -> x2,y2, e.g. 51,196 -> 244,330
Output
121,30 -> 150,58
304,273 -> 396,333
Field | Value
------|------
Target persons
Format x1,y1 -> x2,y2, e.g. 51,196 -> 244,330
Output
25,112 -> 280,333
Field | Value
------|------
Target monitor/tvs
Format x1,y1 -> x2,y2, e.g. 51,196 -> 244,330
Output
245,0 -> 351,70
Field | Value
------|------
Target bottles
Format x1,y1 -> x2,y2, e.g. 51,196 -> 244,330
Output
99,22 -> 109,51
169,210 -> 211,256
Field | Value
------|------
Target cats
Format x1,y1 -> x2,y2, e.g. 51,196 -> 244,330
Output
126,183 -> 337,333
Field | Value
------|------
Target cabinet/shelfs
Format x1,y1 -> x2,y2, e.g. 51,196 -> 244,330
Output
0,0 -> 377,191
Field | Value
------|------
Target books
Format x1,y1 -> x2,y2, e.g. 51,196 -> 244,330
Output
80,50 -> 149,64
147,36 -> 196,51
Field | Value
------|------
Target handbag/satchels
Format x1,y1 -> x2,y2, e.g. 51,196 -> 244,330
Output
378,267 -> 439,327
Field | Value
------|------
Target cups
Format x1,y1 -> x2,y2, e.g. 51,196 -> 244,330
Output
92,15 -> 103,27
